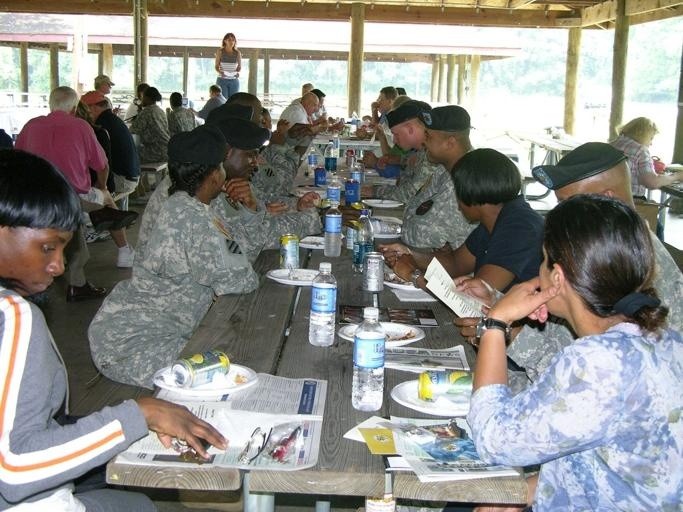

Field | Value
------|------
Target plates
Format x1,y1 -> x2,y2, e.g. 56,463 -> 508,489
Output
264,267 -> 319,287
390,378 -> 474,419
298,236 -> 327,249
336,320 -> 428,349
150,361 -> 259,397
383,272 -> 425,291
362,199 -> 405,209
370,216 -> 404,238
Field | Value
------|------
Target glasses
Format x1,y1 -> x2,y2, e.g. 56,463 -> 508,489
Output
237,426 -> 273,468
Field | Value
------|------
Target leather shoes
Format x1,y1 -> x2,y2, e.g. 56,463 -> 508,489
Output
67,280 -> 108,302
90,207 -> 138,229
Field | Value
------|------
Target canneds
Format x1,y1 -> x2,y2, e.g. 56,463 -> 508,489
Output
346,155 -> 355,166
279,233 -> 300,269
351,171 -> 361,183
171,348 -> 230,389
313,166 -> 325,187
344,179 -> 361,209
362,251 -> 385,293
417,366 -> 474,403
346,219 -> 365,251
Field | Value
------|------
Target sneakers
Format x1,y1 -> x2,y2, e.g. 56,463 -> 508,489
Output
116,247 -> 136,268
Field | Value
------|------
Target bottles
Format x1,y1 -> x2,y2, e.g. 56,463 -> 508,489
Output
321,139 -> 337,175
309,261 -> 337,347
350,111 -> 359,135
324,202 -> 342,257
352,208 -> 374,276
351,308 -> 386,412
326,177 -> 341,204
306,146 -> 319,176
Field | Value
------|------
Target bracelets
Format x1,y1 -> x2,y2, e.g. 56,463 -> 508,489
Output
319,124 -> 322,132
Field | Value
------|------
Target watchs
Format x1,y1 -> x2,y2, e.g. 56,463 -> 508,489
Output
411,268 -> 422,289
474,317 -> 511,342
96,183 -> 108,193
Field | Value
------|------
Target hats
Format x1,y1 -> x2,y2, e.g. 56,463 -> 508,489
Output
419,105 -> 470,131
81,90 -> 105,104
206,105 -> 253,123
220,119 -> 269,148
386,100 -> 431,125
168,122 -> 226,168
532,142 -> 628,191
95,75 -> 116,86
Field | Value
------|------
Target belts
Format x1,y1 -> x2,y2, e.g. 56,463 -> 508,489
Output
124,176 -> 140,183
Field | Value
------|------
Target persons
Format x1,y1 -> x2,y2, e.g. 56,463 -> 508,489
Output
402,105 -> 481,251
226,90 -> 301,167
2,150 -> 229,511
288,89 -> 331,120
371,85 -> 400,130
452,139 -> 682,384
375,148 -> 549,297
128,87 -> 172,193
12,86 -> 139,303
123,83 -> 150,124
279,93 -> 326,136
362,88 -> 409,123
465,191 -> 682,512
94,74 -> 125,119
127,116 -> 323,278
166,92 -> 196,135
261,102 -> 328,146
205,103 -> 292,197
66,100 -> 137,268
1,126 -> 15,150
301,82 -> 327,112
77,90 -> 142,243
609,115 -> 683,242
85,122 -> 261,389
215,33 -> 242,100
358,103 -> 434,179
188,85 -> 228,126
375,95 -> 413,159
355,102 -> 441,201
